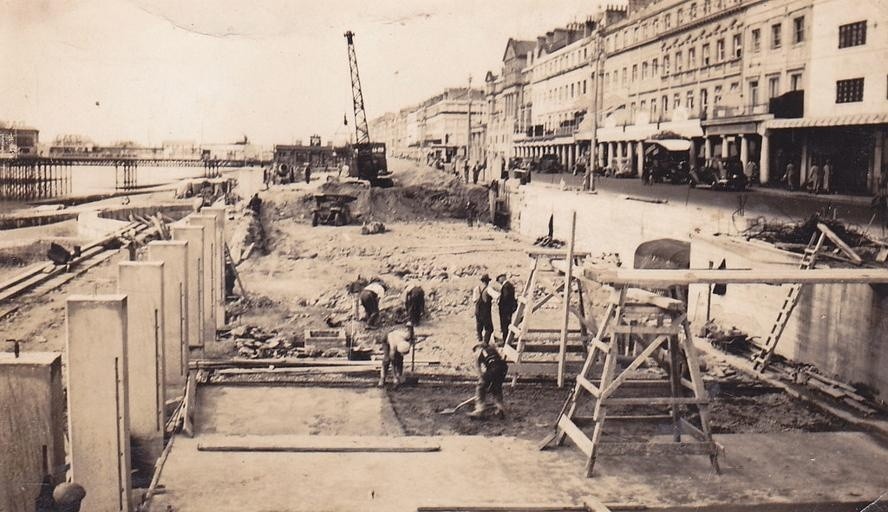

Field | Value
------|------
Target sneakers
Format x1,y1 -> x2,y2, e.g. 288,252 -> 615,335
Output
376,381 -> 385,388
365,325 -> 378,330
466,410 -> 485,417
494,408 -> 504,415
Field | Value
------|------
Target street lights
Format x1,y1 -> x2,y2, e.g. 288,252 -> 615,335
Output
588,47 -> 608,195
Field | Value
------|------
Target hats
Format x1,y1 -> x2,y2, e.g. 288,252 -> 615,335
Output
496,274 -> 506,281
481,274 -> 491,281
397,342 -> 409,352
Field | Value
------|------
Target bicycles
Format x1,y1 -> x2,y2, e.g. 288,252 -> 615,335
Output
641,167 -> 650,185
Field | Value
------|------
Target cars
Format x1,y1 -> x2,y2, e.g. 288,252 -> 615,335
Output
537,154 -> 563,174
615,163 -> 634,179
687,156 -> 749,193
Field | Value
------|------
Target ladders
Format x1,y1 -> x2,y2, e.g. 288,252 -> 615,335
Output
753,232 -> 826,374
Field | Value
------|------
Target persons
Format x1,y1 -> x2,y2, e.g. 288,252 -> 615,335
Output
782,160 -> 796,192
377,328 -> 415,389
462,158 -> 471,183
464,196 -> 477,228
808,160 -> 821,194
745,155 -> 758,189
248,192 -> 263,218
360,280 -> 390,330
494,272 -> 516,346
473,274 -> 503,348
399,283 -> 425,327
471,160 -> 485,184
52,481 -> 87,512
465,342 -> 509,418
821,158 -> 836,194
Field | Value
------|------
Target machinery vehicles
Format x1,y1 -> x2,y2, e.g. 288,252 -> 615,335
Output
341,32 -> 394,188
